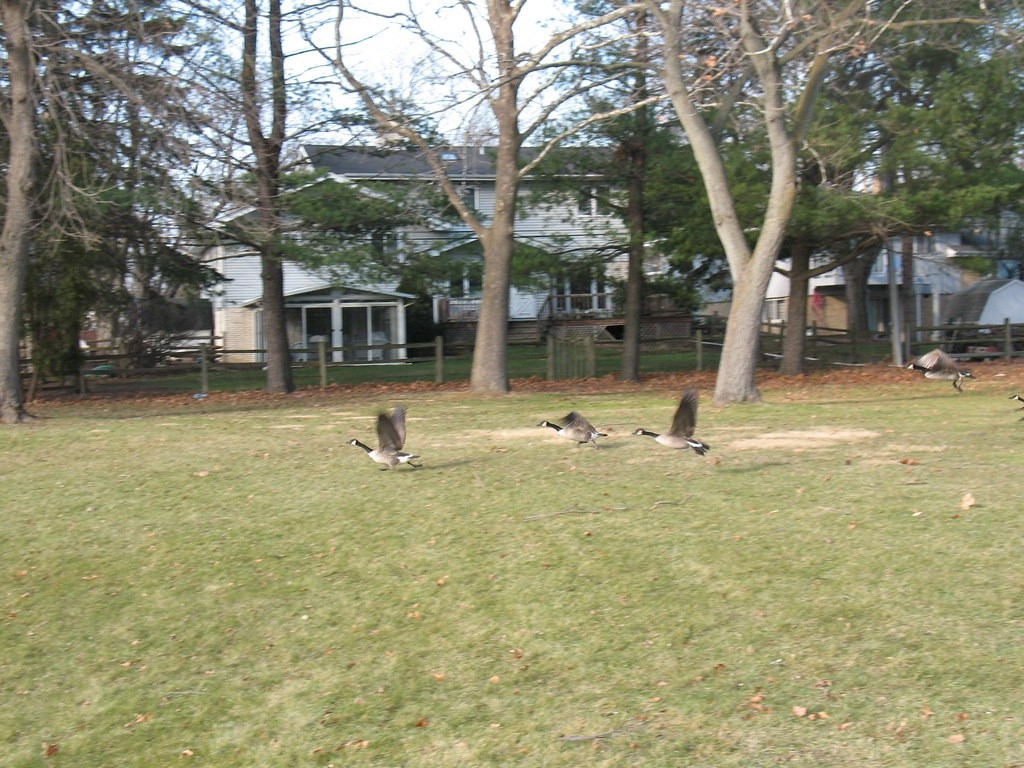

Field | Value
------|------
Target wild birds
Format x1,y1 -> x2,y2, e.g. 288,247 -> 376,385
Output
905,348 -> 976,395
344,404 -> 423,471
1008,395 -> 1024,404
537,409 -> 608,450
631,386 -> 710,458
